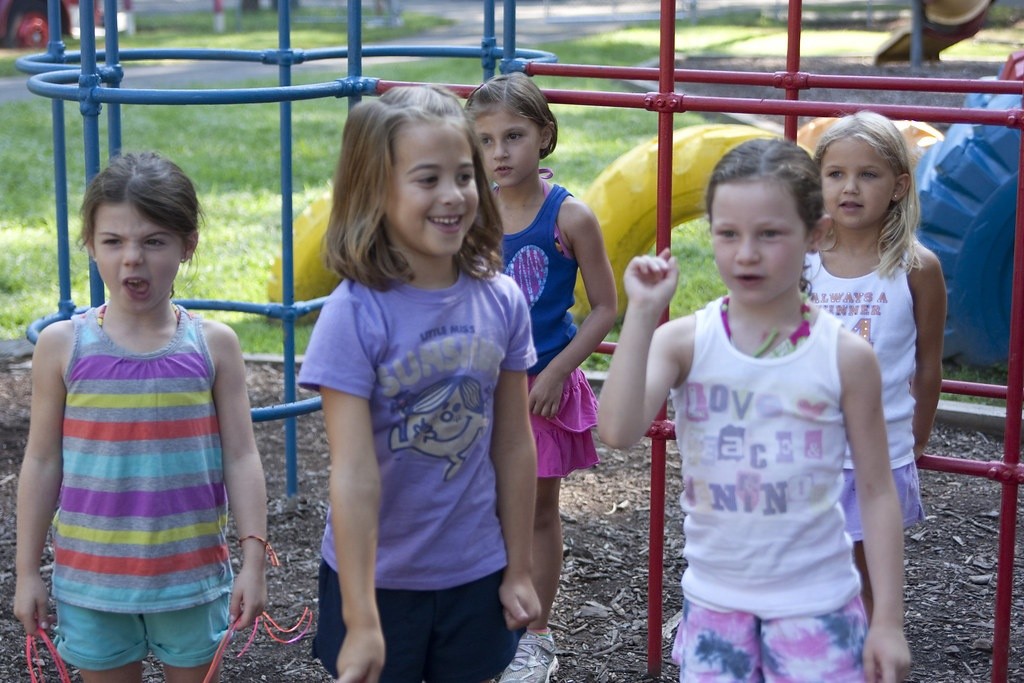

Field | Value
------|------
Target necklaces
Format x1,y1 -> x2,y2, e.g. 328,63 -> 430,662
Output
719,293 -> 810,359
97,300 -> 182,332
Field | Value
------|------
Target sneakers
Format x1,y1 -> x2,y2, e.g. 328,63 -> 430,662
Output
498,629 -> 560,683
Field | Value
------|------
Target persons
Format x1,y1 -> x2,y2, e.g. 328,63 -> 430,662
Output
296,85 -> 543,683
793,108 -> 948,627
462,72 -> 618,683
14,153 -> 271,683
596,139 -> 912,683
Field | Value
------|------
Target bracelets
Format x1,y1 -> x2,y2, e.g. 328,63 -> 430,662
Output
237,535 -> 281,568
